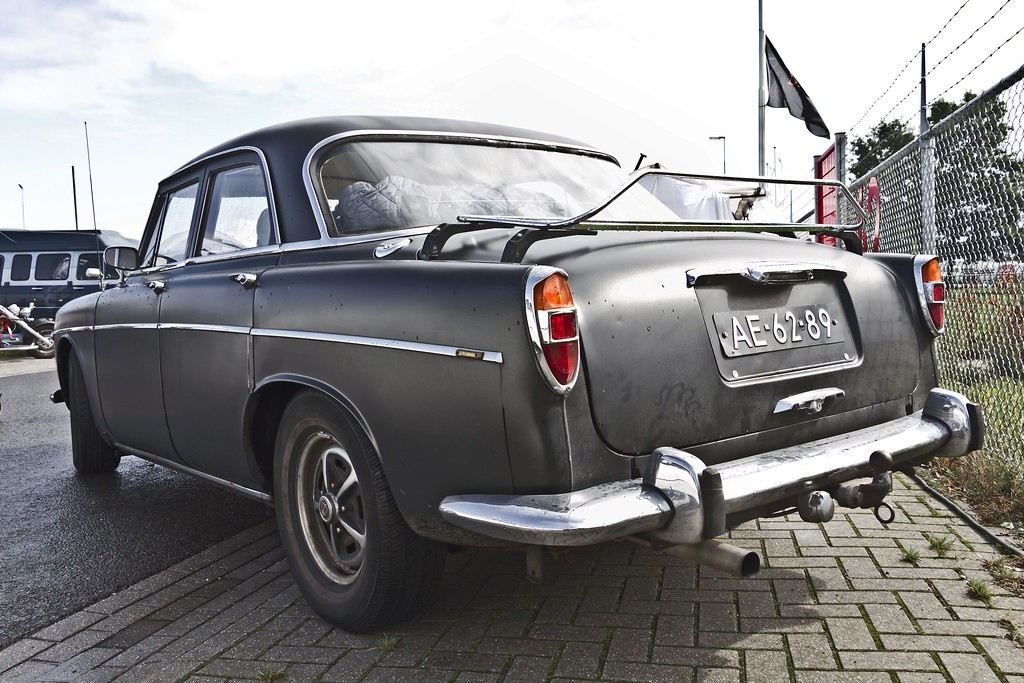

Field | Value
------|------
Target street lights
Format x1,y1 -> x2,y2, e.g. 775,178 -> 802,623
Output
709,137 -> 727,173
18,184 -> 25,229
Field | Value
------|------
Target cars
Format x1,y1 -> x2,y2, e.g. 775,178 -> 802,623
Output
51,115 -> 984,633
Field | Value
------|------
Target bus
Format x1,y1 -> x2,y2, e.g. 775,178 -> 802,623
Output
0,229 -> 140,358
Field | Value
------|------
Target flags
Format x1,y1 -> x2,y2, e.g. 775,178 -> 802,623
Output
764,34 -> 831,140
